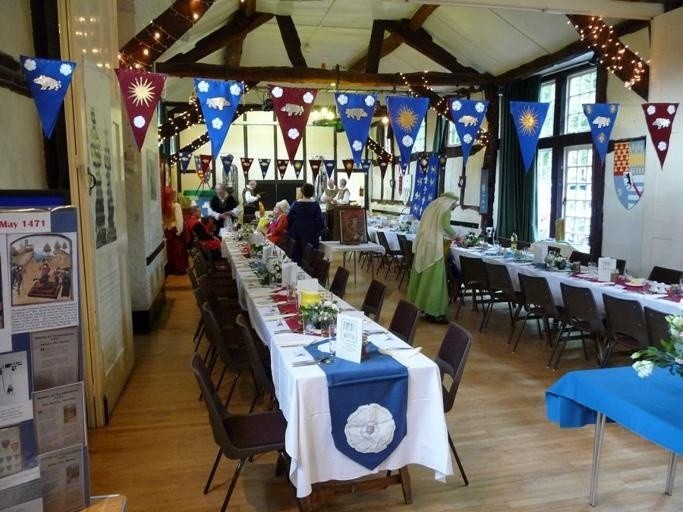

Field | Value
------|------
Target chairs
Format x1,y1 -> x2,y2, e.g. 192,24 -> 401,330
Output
183,213 -> 683,512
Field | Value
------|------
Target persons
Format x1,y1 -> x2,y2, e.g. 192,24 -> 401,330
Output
406,191 -> 460,323
165,178 -> 350,266
12,260 -> 71,297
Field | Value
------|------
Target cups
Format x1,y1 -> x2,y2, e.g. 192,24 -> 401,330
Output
267,248 -> 306,315
300,290 -> 336,354
588,262 -> 597,276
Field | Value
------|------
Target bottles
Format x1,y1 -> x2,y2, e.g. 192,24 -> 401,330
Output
650,280 -> 665,293
510,232 -> 518,249
224,215 -> 237,232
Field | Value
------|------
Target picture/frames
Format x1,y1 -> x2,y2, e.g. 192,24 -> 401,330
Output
338,207 -> 368,244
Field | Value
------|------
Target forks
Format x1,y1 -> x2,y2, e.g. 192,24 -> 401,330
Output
272,327 -> 299,334
341,307 -> 412,351
289,356 -> 340,368
